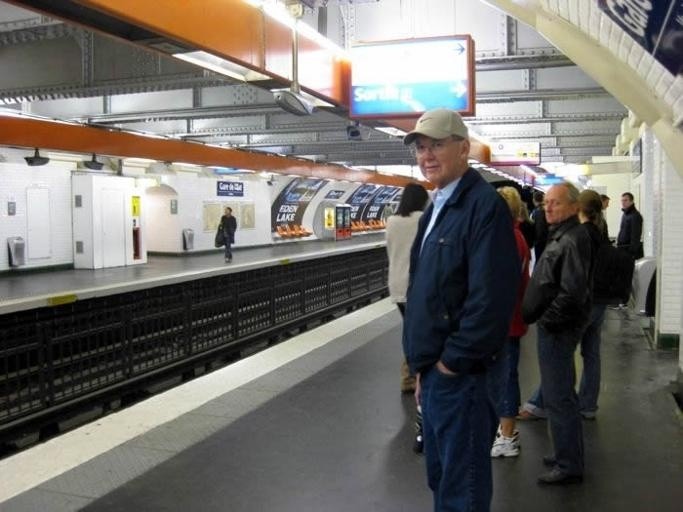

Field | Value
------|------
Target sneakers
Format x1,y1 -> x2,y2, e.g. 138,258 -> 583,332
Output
606,303 -> 629,310
400,357 -> 417,394
516,411 -> 547,421
488,425 -> 522,458
580,409 -> 597,420
536,451 -> 582,485
411,411 -> 424,457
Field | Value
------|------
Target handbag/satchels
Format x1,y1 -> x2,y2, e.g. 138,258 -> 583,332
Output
626,242 -> 644,260
215,224 -> 226,248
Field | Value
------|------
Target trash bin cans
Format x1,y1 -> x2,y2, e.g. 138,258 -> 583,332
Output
182,229 -> 193,249
9,237 -> 24,266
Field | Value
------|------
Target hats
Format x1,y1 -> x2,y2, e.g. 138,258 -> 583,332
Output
402,107 -> 469,148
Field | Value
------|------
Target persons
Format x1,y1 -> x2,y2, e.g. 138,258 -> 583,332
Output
401,108 -> 518,510
490,180 -> 643,486
385,182 -> 431,455
219,206 -> 237,262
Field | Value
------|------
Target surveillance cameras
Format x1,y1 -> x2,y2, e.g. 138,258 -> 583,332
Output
84,161 -> 104,169
270,89 -> 314,117
24,157 -> 49,167
347,126 -> 372,141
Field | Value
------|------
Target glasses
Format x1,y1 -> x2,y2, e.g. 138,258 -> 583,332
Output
410,138 -> 462,157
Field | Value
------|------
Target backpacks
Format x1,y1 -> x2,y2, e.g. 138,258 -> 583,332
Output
583,224 -> 620,307
